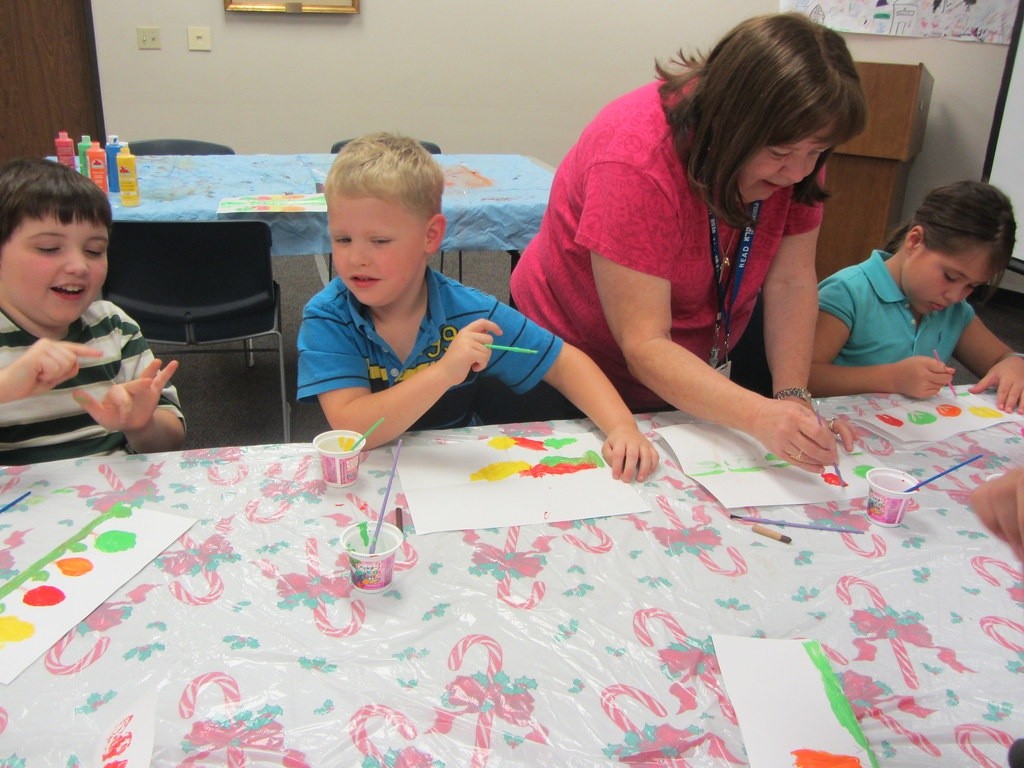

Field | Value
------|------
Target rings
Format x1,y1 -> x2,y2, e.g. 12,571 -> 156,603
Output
828,417 -> 836,431
790,450 -> 802,461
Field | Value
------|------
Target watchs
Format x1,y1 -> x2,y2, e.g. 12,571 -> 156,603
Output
775,387 -> 811,402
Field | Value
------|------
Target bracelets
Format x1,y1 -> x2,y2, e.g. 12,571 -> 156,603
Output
1007,354 -> 1024,356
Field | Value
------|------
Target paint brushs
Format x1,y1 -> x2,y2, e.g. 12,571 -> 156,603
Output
351,417 -> 386,451
903,454 -> 984,491
932,349 -> 960,397
0,491 -> 32,512
483,344 -> 537,353
731,514 -> 864,535
370,439 -> 403,554
816,408 -> 848,488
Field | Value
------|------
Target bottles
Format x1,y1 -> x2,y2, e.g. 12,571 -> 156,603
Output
115,147 -> 140,207
54,131 -> 77,171
105,135 -> 123,194
86,141 -> 110,198
77,135 -> 92,178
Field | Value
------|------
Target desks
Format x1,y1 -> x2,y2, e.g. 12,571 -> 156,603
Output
0,380 -> 1023,766
44,148 -> 554,311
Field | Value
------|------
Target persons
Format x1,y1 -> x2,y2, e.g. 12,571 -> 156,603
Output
296,133 -> 659,483
510,12 -> 866,474
0,158 -> 186,466
806,180 -> 1024,416
969,465 -> 1024,768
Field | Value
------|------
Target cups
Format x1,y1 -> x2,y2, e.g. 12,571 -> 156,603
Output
312,430 -> 366,487
339,520 -> 404,593
866,467 -> 921,528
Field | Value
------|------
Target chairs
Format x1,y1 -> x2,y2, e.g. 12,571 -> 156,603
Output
95,140 -> 445,444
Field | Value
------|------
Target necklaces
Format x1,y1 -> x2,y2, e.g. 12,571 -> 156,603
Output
717,223 -> 737,284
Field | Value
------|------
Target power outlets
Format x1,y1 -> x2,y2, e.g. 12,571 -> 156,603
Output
137,28 -> 162,49
